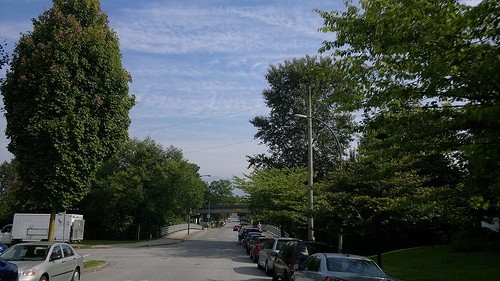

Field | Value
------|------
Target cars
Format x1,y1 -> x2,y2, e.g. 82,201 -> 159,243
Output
258,237 -> 303,275
233,220 -> 266,254
0,241 -> 83,281
270,240 -> 335,281
250,237 -> 272,263
289,252 -> 394,281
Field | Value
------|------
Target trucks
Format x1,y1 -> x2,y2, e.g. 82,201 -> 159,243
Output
0,214 -> 85,246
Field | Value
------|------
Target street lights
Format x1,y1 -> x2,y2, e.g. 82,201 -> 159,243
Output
293,114 -> 343,253
